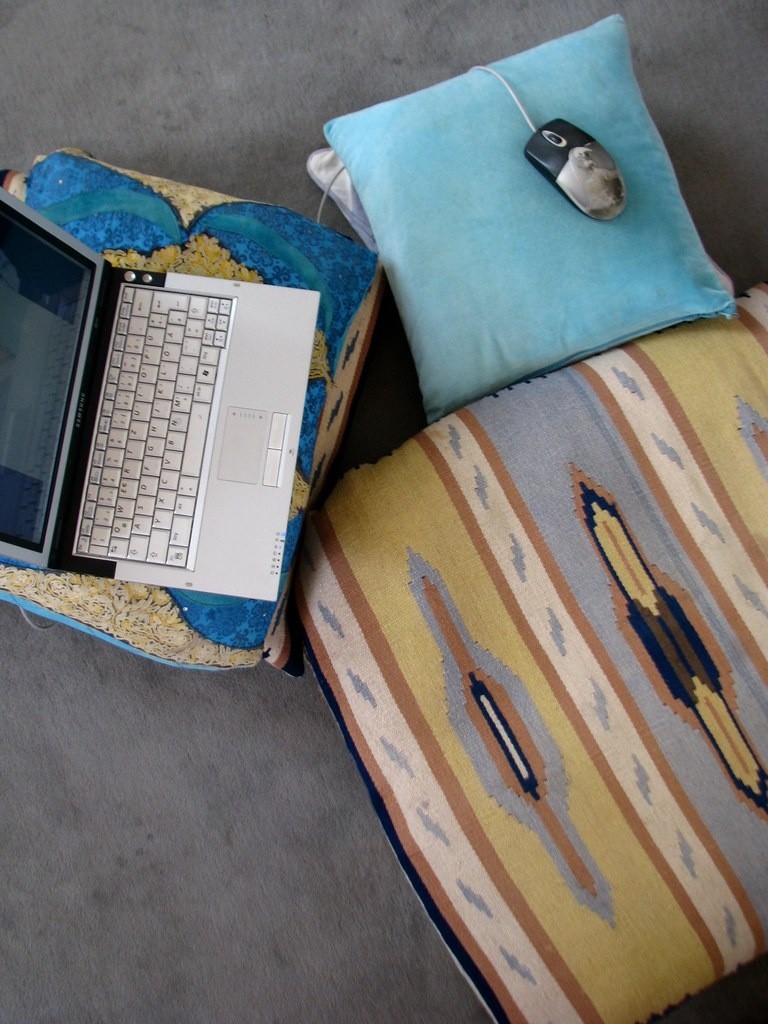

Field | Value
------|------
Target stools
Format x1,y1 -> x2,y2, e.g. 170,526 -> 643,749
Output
0,147 -> 377,678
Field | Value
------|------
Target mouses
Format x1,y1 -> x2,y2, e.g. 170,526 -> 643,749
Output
525,117 -> 628,221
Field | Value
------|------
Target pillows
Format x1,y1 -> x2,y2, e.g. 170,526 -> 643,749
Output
305,12 -> 735,426
296,281 -> 768,1024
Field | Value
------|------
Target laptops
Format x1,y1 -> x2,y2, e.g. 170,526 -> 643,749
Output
0,189 -> 322,601
